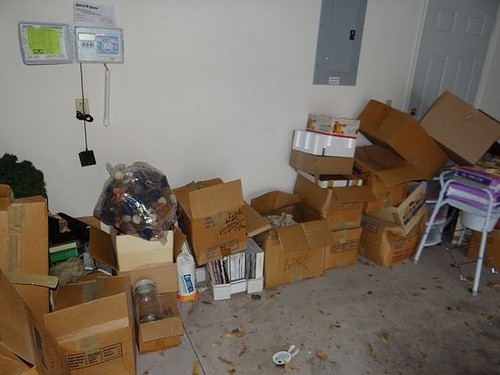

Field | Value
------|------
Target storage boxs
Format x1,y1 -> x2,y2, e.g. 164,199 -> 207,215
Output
0,91 -> 500,375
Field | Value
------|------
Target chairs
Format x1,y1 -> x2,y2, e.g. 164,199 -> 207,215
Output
413,170 -> 500,297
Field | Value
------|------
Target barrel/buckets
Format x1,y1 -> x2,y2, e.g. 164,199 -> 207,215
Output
461,208 -> 500,231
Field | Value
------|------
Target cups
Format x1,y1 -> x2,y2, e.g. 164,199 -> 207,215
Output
133,279 -> 160,321
